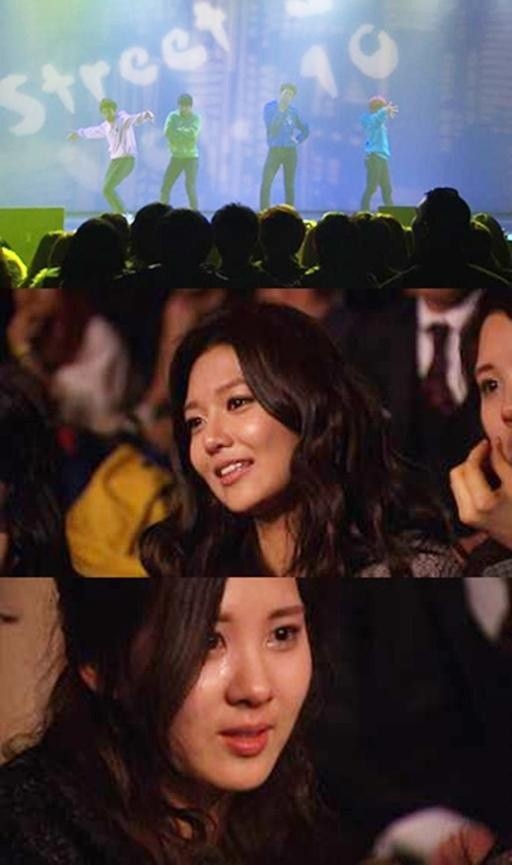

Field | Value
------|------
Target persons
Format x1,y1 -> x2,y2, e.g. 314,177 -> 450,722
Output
159,92 -> 202,210
2,181 -> 511,865
361,95 -> 399,212
65,97 -> 154,216
259,84 -> 310,212
